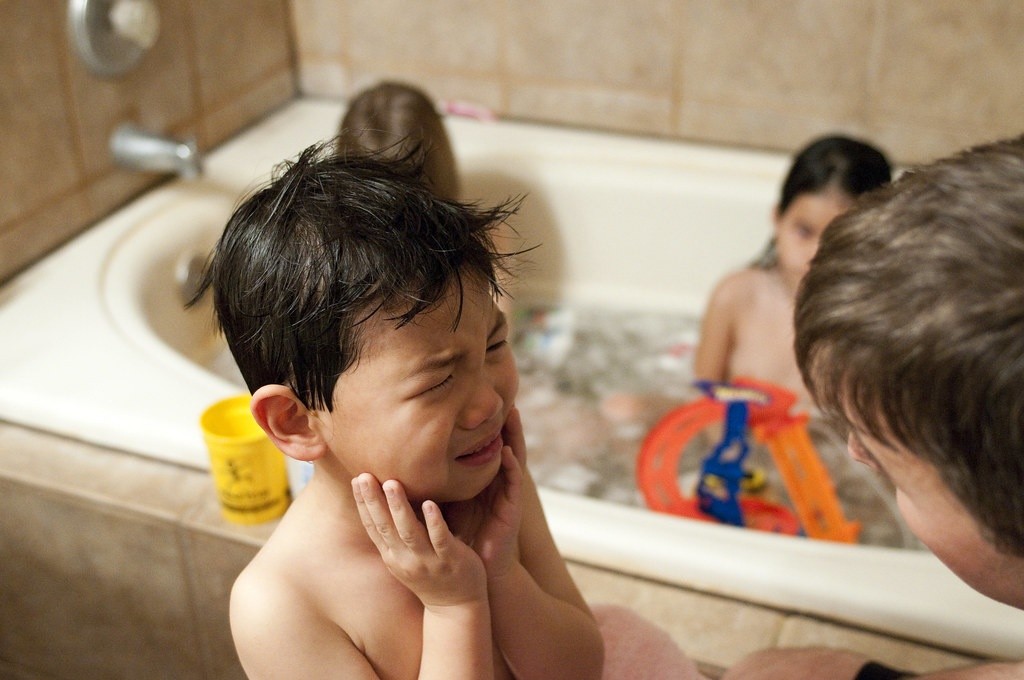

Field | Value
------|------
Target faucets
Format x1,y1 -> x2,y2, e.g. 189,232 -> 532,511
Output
107,121 -> 204,182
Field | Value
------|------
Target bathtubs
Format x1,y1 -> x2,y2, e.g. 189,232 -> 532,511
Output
0,95 -> 1024,679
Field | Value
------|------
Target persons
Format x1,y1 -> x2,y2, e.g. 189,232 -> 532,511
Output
584,132 -> 1024,679
341,85 -> 513,340
694,134 -> 893,408
185,134 -> 605,679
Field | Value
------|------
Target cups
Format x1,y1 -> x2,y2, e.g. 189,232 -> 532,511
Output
198,394 -> 291,528
